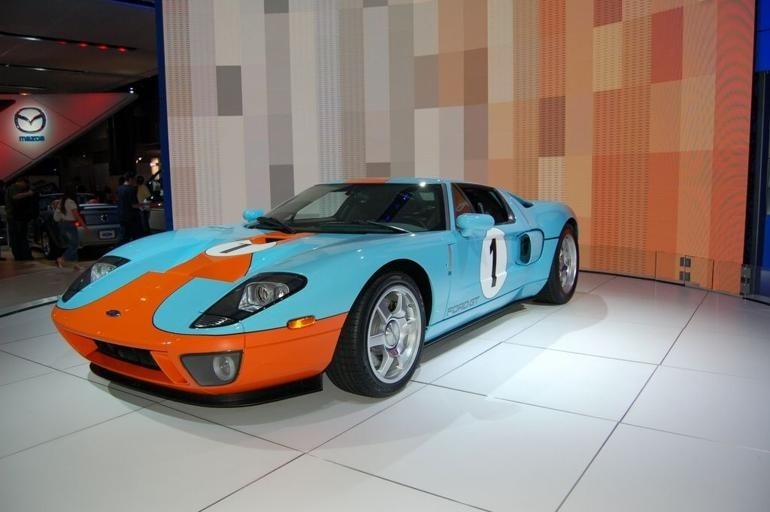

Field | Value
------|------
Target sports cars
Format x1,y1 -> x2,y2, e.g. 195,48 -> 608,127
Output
50,176 -> 580,408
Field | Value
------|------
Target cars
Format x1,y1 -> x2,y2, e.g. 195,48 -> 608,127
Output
0,194 -> 121,259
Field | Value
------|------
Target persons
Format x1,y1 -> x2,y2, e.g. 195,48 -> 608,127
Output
53,181 -> 89,271
5,174 -> 34,260
115,176 -> 126,202
117,171 -> 152,243
136,175 -> 154,236
83,182 -> 113,206
